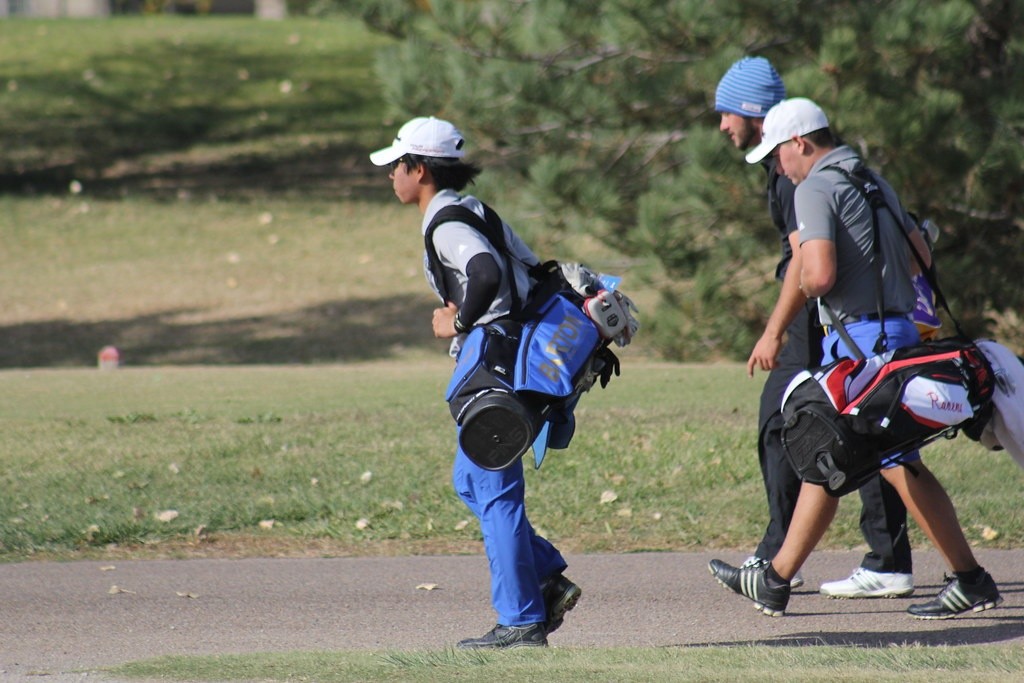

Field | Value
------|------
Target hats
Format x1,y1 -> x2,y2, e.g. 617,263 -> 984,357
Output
369,115 -> 465,166
715,56 -> 785,117
744,97 -> 829,165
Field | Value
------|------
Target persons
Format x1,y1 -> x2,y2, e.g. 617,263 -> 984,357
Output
708,98 -> 1003,621
714,56 -> 914,600
369,115 -> 582,651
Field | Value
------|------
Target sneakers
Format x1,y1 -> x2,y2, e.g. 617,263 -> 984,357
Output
709,558 -> 791,617
536,570 -> 581,637
458,612 -> 548,652
740,550 -> 804,588
818,563 -> 914,601
907,569 -> 1001,620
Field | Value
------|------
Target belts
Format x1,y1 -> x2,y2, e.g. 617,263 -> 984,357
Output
823,310 -> 907,336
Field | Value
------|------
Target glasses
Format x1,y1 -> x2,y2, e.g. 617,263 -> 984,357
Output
391,157 -> 410,169
